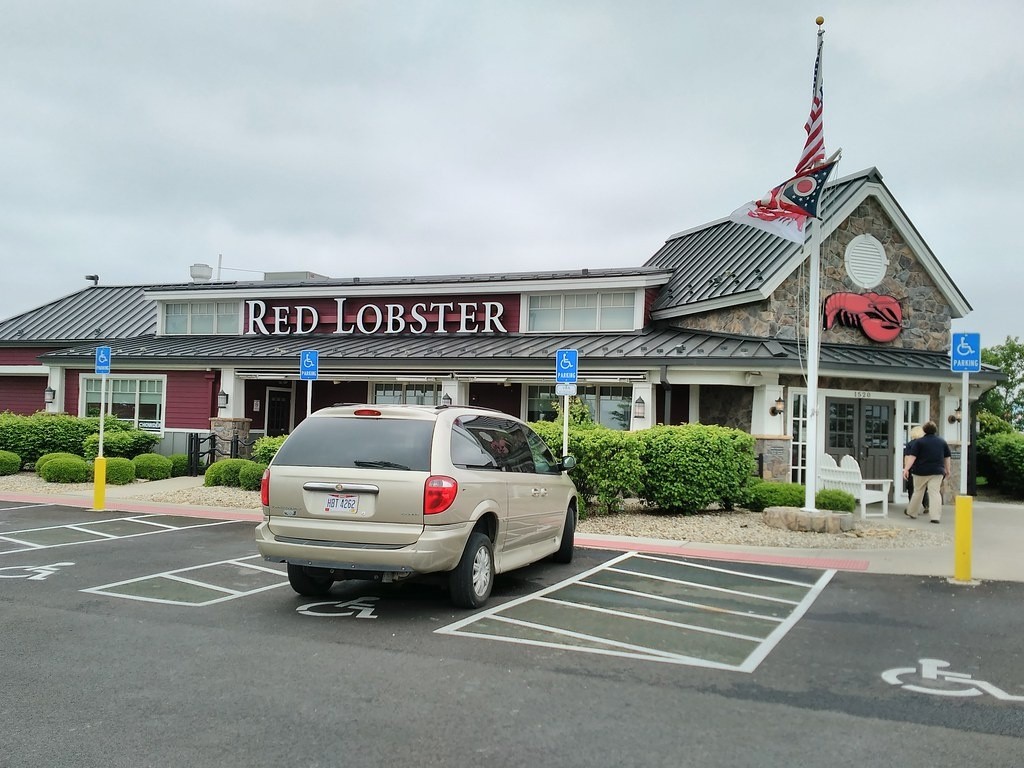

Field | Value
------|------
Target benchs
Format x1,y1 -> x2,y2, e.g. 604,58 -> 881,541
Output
818,453 -> 894,518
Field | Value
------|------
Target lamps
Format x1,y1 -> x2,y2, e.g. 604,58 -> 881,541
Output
217,389 -> 227,408
769,396 -> 785,416
634,396 -> 645,418
44,387 -> 56,403
948,406 -> 962,424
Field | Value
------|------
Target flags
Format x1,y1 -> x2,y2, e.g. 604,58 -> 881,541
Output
728,25 -> 843,247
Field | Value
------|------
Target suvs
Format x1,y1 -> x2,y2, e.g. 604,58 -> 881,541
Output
254,403 -> 581,609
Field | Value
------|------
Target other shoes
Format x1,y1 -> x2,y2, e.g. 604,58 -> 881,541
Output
931,520 -> 940,523
923,509 -> 929,514
904,509 -> 917,519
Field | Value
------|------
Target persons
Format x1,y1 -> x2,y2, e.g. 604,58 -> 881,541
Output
903,419 -> 952,524
